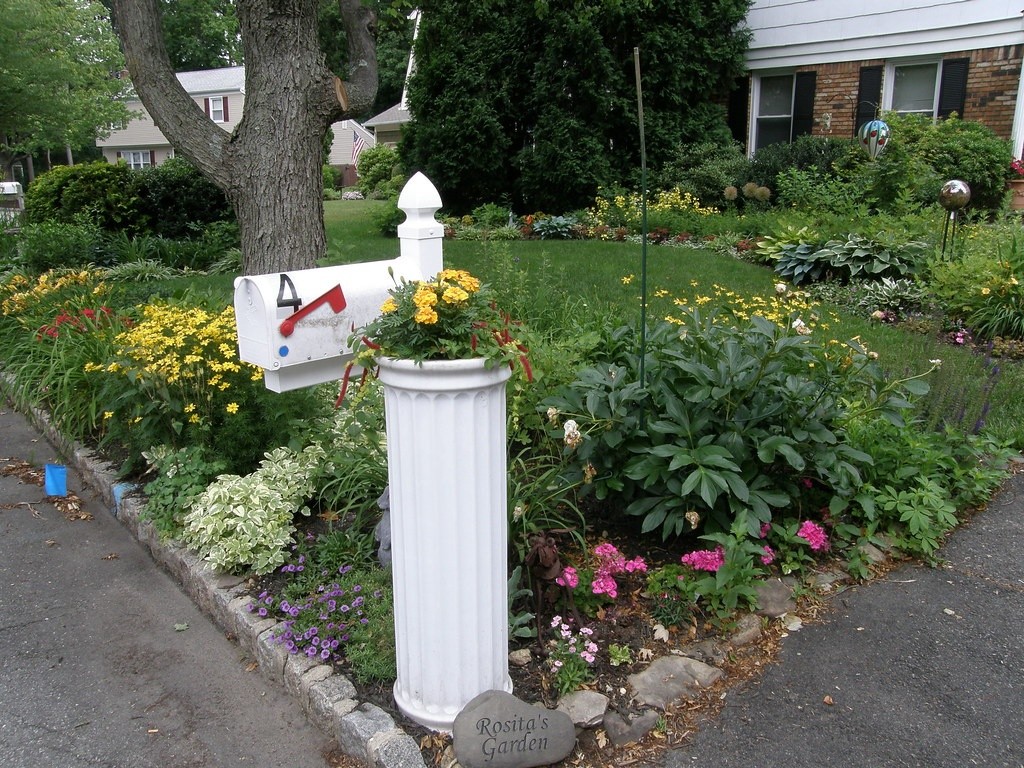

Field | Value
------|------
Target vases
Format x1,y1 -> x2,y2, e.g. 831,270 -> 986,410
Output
1006,179 -> 1024,211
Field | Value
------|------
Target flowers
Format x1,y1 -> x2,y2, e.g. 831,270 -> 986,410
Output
1010,155 -> 1024,179
334,264 -> 533,430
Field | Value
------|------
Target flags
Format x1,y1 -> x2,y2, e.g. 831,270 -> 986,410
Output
352,130 -> 365,170
